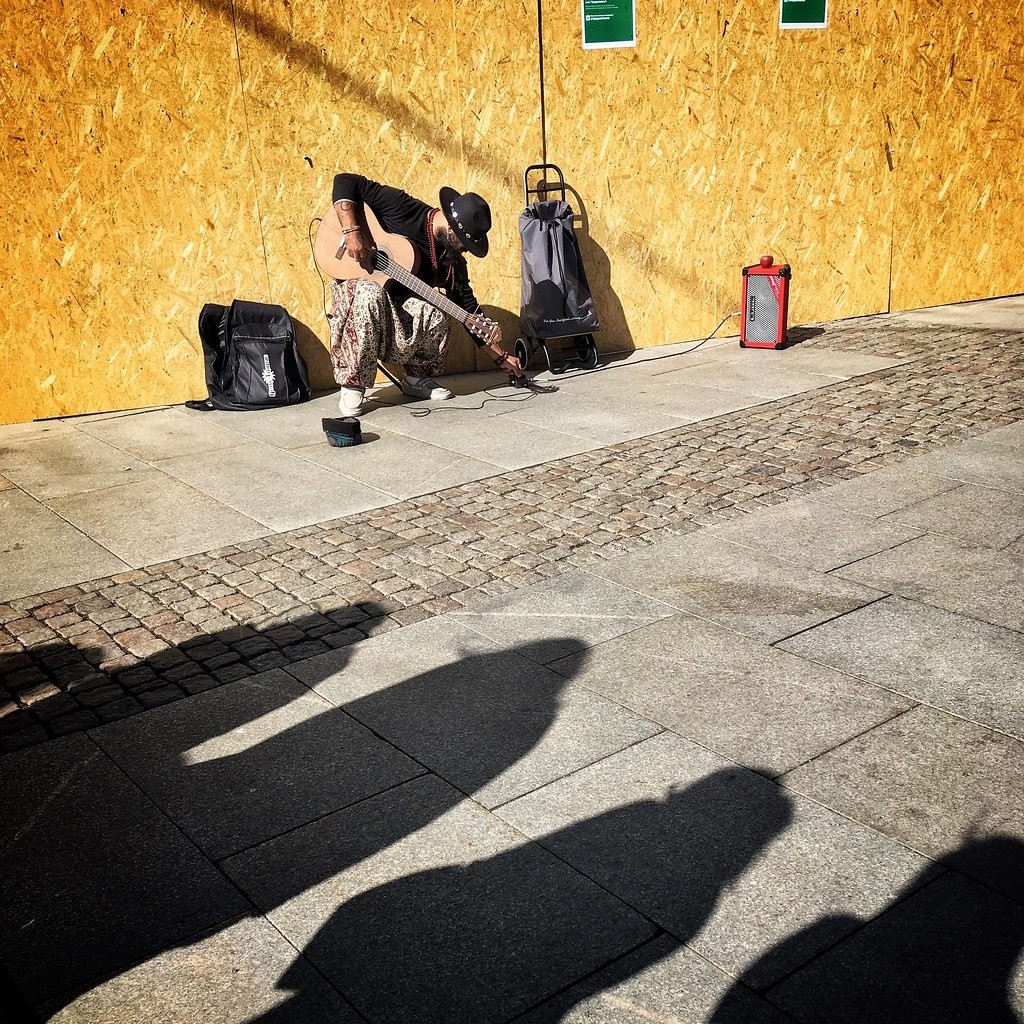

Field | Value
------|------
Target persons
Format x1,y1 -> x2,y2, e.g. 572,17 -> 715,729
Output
327,174 -> 521,417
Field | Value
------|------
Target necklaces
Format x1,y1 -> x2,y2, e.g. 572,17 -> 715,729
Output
426,207 -> 455,295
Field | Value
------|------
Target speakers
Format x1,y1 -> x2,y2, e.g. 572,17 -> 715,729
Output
739,265 -> 790,349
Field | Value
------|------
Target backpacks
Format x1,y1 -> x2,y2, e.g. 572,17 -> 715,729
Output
184,299 -> 313,412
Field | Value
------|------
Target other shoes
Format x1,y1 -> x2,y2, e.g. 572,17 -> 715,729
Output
339,385 -> 365,417
402,376 -> 454,401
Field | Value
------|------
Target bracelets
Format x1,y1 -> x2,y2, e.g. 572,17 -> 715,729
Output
495,350 -> 508,366
342,225 -> 360,234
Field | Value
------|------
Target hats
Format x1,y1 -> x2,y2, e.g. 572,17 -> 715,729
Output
439,186 -> 492,258
